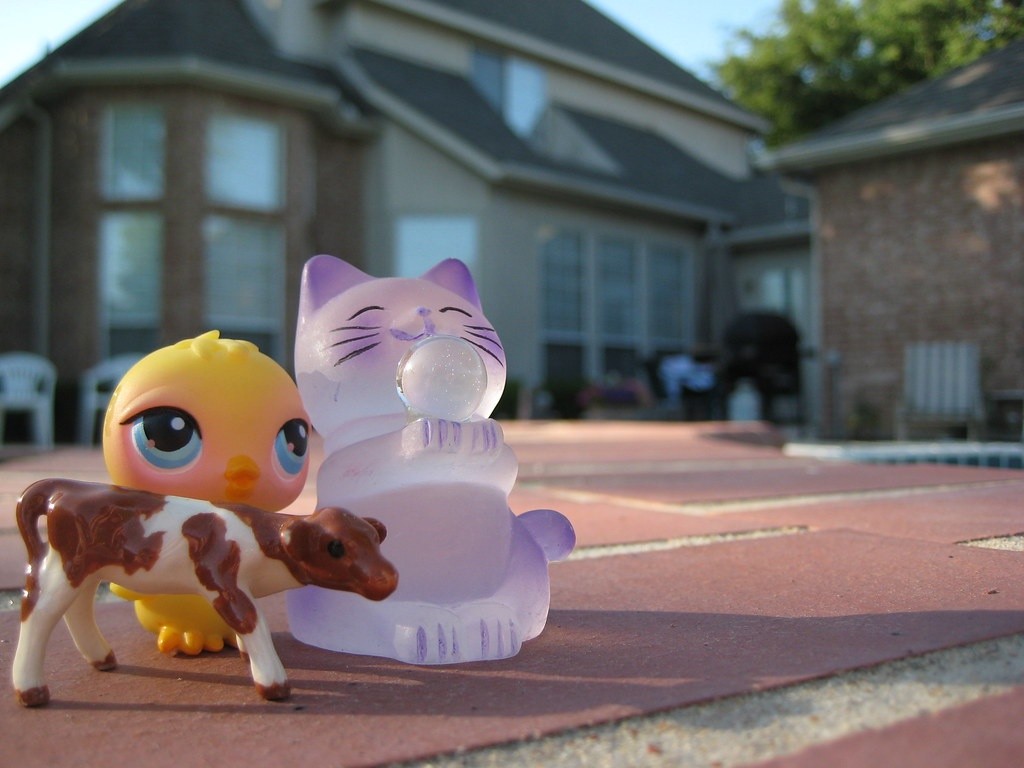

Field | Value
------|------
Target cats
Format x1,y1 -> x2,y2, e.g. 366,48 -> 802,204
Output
285,255 -> 574,665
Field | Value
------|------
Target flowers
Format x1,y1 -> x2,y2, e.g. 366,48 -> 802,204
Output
571,374 -> 651,408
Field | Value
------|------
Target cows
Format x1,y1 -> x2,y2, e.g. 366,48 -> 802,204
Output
11,477 -> 391,703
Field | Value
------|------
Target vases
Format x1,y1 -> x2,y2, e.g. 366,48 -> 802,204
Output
585,403 -> 662,421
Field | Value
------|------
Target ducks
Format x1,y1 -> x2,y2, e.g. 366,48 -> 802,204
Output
101,329 -> 313,655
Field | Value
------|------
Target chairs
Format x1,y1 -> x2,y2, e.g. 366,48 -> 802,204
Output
0,352 -> 59,450
894,340 -> 990,445
81,353 -> 145,443
